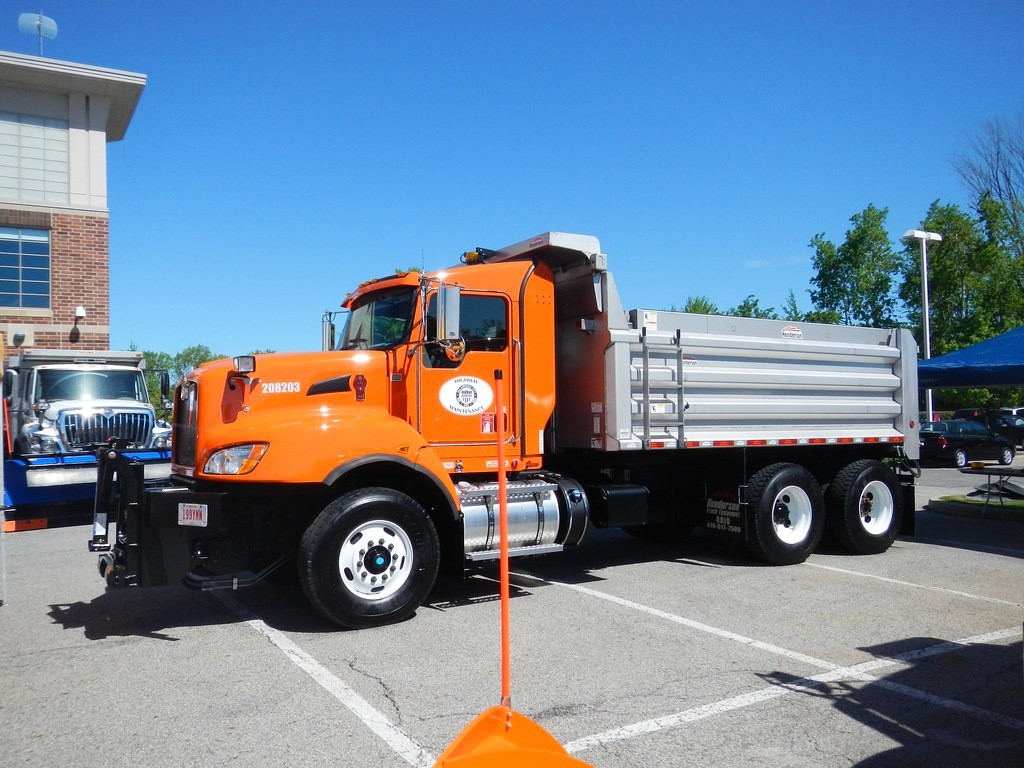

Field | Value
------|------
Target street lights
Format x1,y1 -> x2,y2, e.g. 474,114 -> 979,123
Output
903,229 -> 942,431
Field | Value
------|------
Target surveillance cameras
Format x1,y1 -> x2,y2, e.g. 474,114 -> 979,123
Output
75,306 -> 86,320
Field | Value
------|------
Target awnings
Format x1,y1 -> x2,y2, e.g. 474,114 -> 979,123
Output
916,324 -> 1024,391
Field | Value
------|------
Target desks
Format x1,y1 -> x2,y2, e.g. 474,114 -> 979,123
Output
957,467 -> 1024,527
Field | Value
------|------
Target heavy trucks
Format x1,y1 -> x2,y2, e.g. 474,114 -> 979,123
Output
2,347 -> 177,519
87,230 -> 920,629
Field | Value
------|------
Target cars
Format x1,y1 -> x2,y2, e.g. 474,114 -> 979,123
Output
952,407 -> 1024,449
920,418 -> 1016,468
